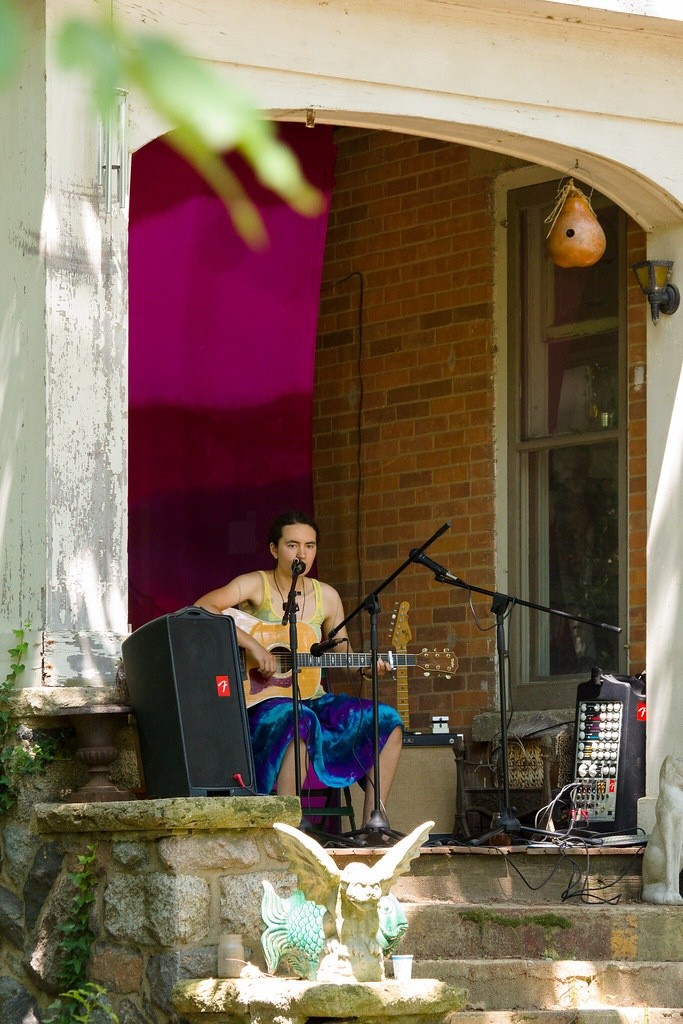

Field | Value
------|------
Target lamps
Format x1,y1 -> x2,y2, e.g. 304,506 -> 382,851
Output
632,259 -> 680,326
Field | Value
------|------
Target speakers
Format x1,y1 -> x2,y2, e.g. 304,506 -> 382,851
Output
122,606 -> 259,798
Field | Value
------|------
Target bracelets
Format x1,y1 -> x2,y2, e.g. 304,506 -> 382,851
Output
357,667 -> 372,681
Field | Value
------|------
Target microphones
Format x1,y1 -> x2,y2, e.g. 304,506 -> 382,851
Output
409,548 -> 458,581
292,557 -> 306,574
310,638 -> 343,657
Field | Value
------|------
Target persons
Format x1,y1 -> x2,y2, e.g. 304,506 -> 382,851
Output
193,513 -> 404,845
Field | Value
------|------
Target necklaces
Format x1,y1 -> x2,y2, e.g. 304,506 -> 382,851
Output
274,568 -> 306,622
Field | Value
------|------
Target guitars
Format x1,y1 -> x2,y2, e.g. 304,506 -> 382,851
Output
219,607 -> 460,710
387,600 -> 412,735
